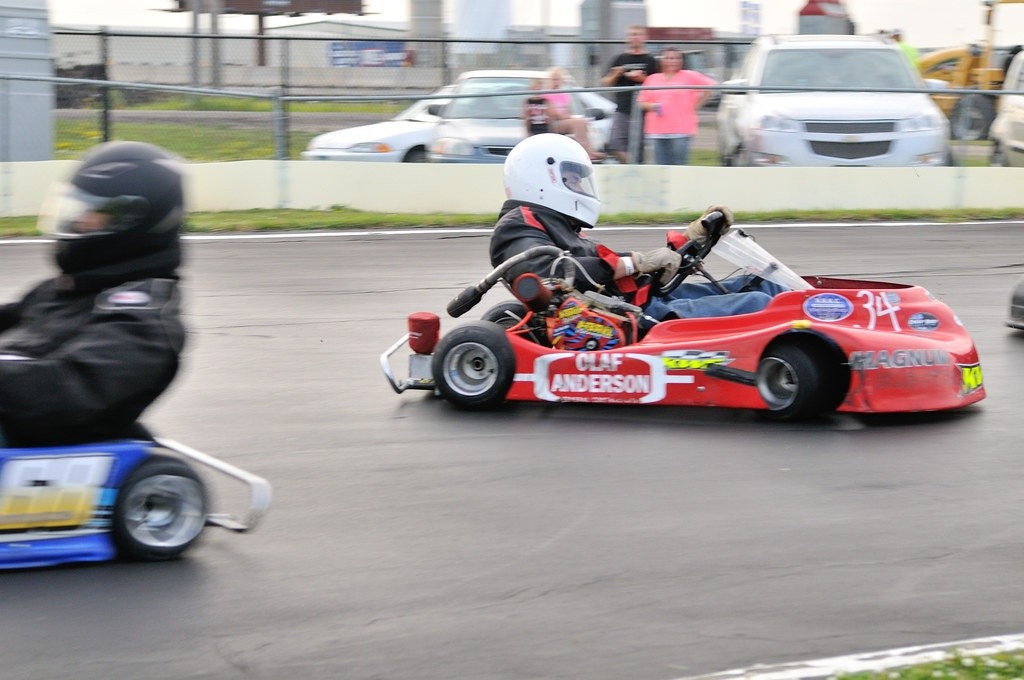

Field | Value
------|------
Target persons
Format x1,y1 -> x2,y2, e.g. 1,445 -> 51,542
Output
491,133 -> 793,348
524,27 -> 717,163
0,139 -> 190,448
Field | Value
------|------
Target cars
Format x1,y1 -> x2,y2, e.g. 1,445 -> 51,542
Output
989,49 -> 1024,167
303,80 -> 625,174
426,67 -> 608,168
905,44 -> 1016,143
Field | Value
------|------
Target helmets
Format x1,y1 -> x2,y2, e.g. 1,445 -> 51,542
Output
38,141 -> 187,292
503,133 -> 601,228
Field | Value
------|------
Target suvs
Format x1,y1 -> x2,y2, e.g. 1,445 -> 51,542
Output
714,33 -> 956,168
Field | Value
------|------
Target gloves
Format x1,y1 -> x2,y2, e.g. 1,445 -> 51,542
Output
684,204 -> 734,245
631,248 -> 682,285
